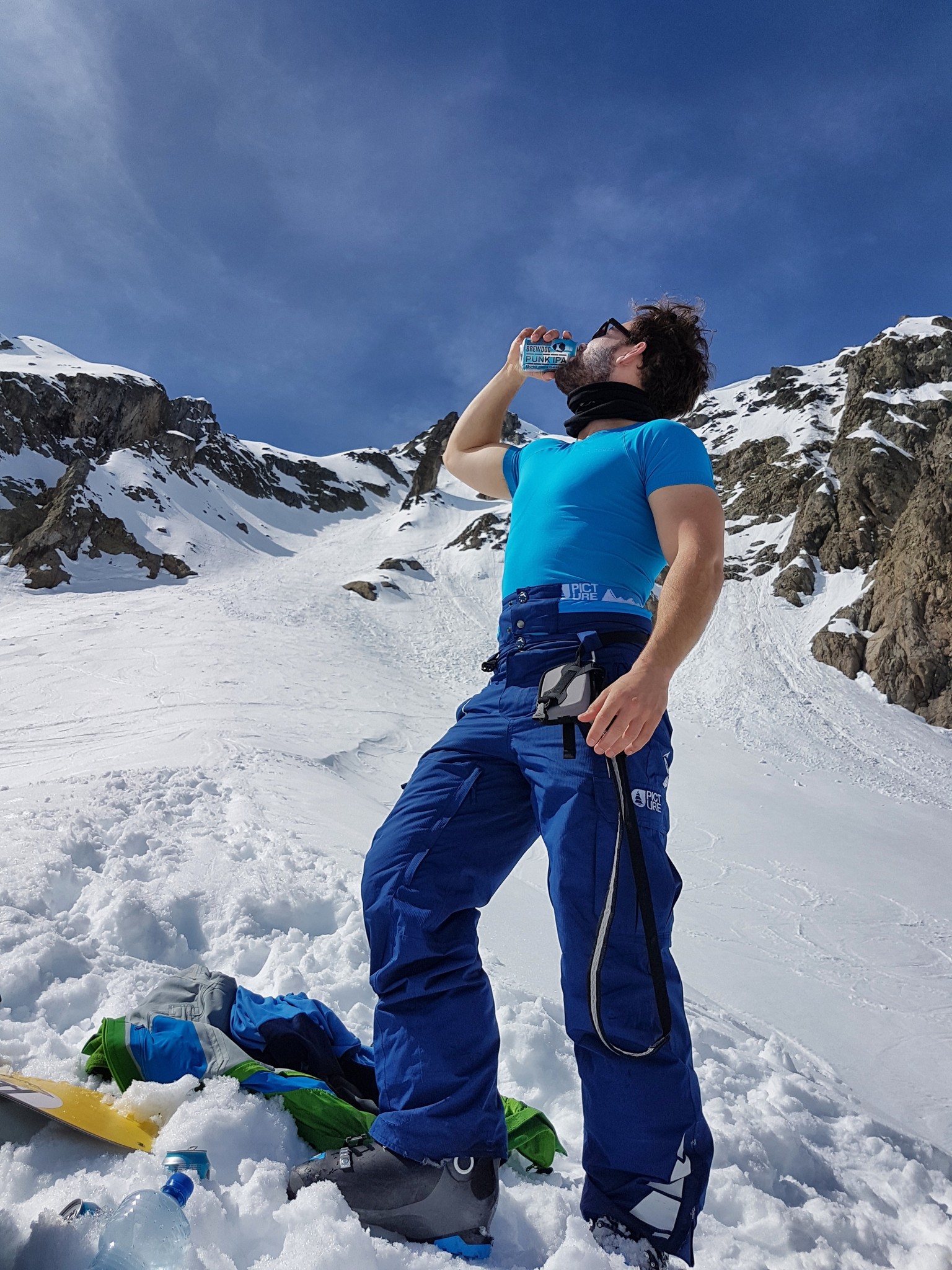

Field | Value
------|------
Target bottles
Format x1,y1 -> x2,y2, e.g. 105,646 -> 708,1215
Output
85,1172 -> 195,1270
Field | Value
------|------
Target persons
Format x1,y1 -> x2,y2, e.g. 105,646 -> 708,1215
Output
282,289 -> 727,1269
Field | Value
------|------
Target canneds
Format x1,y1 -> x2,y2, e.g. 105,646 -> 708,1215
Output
161,1146 -> 210,1184
519,337 -> 578,373
57,1198 -> 104,1223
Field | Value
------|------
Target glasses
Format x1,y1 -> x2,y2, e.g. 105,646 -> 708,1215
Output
592,318 -> 647,366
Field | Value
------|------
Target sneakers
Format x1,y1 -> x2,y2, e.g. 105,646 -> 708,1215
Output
285,1138 -> 501,1238
